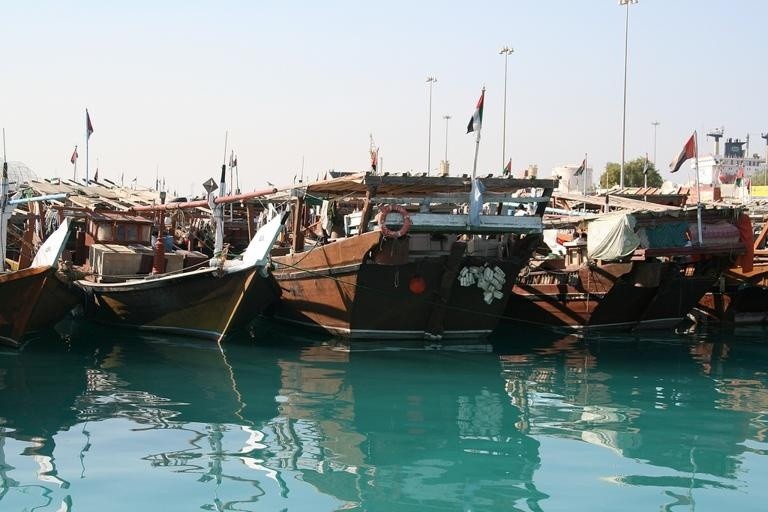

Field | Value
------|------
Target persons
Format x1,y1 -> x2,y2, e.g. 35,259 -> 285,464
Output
152,224 -> 213,257
514,204 -> 528,216
573,228 -> 588,240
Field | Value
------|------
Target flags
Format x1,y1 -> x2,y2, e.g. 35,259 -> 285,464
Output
229,153 -> 234,167
575,160 -> 585,177
465,95 -> 484,134
87,113 -> 92,139
371,151 -> 376,172
233,157 -> 237,167
504,160 -> 512,175
666,135 -> 697,172
734,166 -> 744,187
71,148 -> 79,164
643,157 -> 650,174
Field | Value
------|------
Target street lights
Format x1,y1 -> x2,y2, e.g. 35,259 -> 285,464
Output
618,0 -> 637,190
425,74 -> 438,176
441,112 -> 453,176
497,45 -> 515,175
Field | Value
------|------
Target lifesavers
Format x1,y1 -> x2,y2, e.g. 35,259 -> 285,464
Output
380,205 -> 410,239
